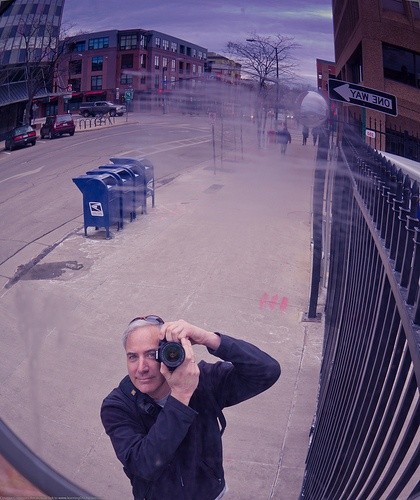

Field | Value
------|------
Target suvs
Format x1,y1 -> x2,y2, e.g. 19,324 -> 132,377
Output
40,114 -> 76,140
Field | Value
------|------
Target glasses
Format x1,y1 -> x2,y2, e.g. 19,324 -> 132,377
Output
129,314 -> 165,325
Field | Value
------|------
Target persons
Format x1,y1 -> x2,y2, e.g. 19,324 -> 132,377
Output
100,315 -> 281,500
302,126 -> 309,145
311,128 -> 319,146
279,127 -> 292,155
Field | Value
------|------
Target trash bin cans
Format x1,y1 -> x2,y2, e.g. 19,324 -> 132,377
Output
72,151 -> 156,240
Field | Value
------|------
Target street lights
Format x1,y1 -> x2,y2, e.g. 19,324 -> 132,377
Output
162,57 -> 184,114
246,38 -> 279,120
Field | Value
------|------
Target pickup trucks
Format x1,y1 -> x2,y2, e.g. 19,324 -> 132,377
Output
79,100 -> 127,117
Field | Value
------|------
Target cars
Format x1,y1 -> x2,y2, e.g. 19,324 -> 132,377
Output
4,125 -> 37,151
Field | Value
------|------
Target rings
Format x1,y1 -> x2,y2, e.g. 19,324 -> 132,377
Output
192,361 -> 195,363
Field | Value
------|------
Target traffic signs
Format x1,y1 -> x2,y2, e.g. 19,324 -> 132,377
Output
328,78 -> 398,118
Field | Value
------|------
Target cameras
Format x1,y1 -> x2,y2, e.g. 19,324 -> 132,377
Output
156,338 -> 185,372
